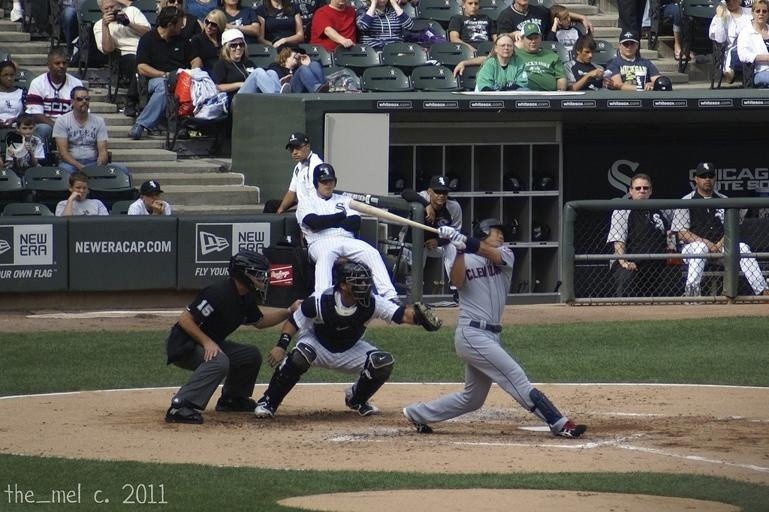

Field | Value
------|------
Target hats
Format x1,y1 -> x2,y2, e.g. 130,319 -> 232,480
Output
618,29 -> 640,44
221,29 -> 243,45
430,176 -> 451,191
520,23 -> 541,36
285,132 -> 309,148
140,179 -> 164,194
5,131 -> 28,158
278,41 -> 306,54
696,162 -> 715,176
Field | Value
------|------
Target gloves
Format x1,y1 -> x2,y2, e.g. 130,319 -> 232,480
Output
534,223 -> 549,240
438,227 -> 456,238
449,232 -> 466,252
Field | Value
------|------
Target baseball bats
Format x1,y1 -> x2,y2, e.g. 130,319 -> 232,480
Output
349,199 -> 439,233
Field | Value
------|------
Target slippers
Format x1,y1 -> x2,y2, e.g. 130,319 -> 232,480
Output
674,53 -> 687,60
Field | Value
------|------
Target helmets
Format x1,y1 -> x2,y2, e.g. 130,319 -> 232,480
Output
229,251 -> 270,304
313,163 -> 337,187
332,257 -> 372,304
504,175 -> 524,191
450,174 -> 464,190
473,218 -> 506,240
535,175 -> 554,191
389,175 -> 409,192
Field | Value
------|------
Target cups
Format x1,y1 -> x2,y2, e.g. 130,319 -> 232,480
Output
636,73 -> 648,92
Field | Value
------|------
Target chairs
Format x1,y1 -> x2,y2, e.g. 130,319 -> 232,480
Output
80,167 -> 135,201
0,129 -> 18,156
407,67 -> 463,92
360,66 -> 414,91
591,40 -> 614,65
463,64 -> 481,91
137,66 -> 163,135
334,45 -> 379,77
427,43 -> 478,64
298,44 -> 332,67
709,40 -> 727,89
22,0 -> 53,32
419,0 -> 463,19
278,216 -> 349,296
71,0 -> 109,80
383,42 -> 427,66
246,44 -> 277,68
49,0 -> 78,48
165,70 -> 228,158
24,167 -> 69,189
0,167 -> 22,191
478,42 -> 494,56
110,201 -> 136,215
572,20 -> 592,41
648,0 -> 674,50
679,0 -> 716,72
91,20 -> 131,100
15,68 -> 35,90
479,0 -> 509,21
743,62 -> 755,87
4,203 -> 53,217
323,68 -> 361,93
407,20 -> 446,43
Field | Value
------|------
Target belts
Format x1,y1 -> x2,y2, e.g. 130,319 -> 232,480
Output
471,320 -> 503,333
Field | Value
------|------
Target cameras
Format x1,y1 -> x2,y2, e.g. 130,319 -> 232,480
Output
108,8 -> 131,25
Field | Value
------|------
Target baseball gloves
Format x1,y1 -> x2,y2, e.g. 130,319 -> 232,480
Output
414,301 -> 442,332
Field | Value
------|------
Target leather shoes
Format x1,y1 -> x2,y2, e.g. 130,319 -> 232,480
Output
124,103 -> 136,116
315,81 -> 330,93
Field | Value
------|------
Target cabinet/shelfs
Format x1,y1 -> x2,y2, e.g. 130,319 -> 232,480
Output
324,112 -> 565,301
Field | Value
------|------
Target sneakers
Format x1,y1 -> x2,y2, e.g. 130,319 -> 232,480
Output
392,298 -> 406,307
450,287 -> 460,303
10,9 -> 23,21
216,397 -> 257,412
280,83 -> 291,93
551,419 -> 587,437
344,395 -> 378,415
403,409 -> 434,433
129,125 -> 142,139
724,69 -> 735,82
166,407 -> 204,424
256,396 -> 274,419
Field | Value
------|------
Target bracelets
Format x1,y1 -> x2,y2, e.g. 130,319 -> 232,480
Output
287,307 -> 292,314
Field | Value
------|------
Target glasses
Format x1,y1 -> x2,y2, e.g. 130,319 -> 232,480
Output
168,0 -> 183,4
433,190 -> 448,195
204,19 -> 217,27
756,9 -> 768,14
632,186 -> 652,191
699,174 -> 716,179
229,42 -> 245,48
288,144 -> 307,150
74,96 -> 91,102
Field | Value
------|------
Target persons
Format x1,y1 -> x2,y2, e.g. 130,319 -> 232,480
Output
219,0 -> 261,44
546,4 -> 593,61
449,0 -> 498,58
59,0 -> 78,65
267,42 -> 329,93
401,219 -> 588,440
263,131 -> 324,215
606,29 -> 662,91
52,86 -> 130,177
606,174 -> 685,297
474,34 -> 532,91
24,49 -> 91,167
93,0 -> 151,117
10,0 -> 24,22
670,163 -> 769,297
210,28 -> 291,115
563,34 -> 622,91
355,0 -> 429,66
0,158 -> 4,170
254,262 -> 442,419
310,0 -> 359,53
453,22 -> 567,91
255,0 -> 304,48
155,0 -> 204,54
296,163 -> 406,310
190,8 -> 227,68
165,249 -> 304,425
128,6 -> 203,139
659,0 -> 699,60
0,60 -> 28,128
128,179 -> 171,215
709,0 -> 754,84
55,173 -> 109,216
4,115 -> 45,174
496,0 -> 594,48
737,0 -> 769,88
398,175 -> 463,304
